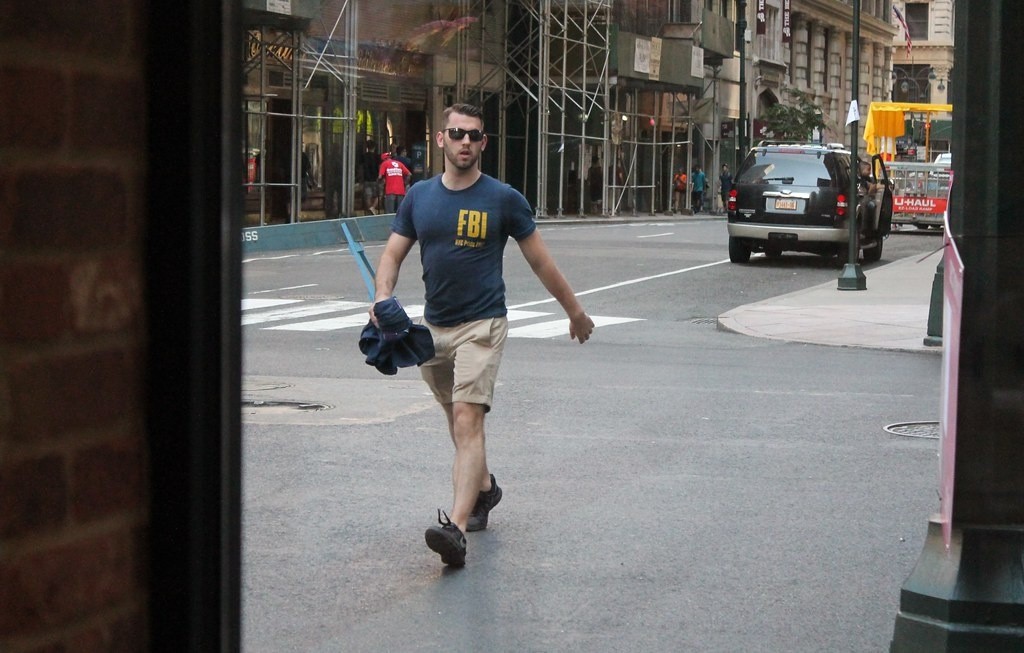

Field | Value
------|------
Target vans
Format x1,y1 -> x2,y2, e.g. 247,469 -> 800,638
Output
875,161 -> 952,229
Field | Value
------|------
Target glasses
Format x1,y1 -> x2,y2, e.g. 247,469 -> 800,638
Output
442,128 -> 485,142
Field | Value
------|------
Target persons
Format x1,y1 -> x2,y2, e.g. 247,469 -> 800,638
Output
857,161 -> 885,193
377,152 -> 412,214
718,163 -> 734,213
588,156 -> 603,216
391,145 -> 414,214
358,140 -> 383,215
369,102 -> 596,567
608,158 -> 625,217
301,152 -> 311,204
691,164 -> 706,214
672,167 -> 687,214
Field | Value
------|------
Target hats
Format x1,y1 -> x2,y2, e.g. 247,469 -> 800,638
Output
380,153 -> 392,160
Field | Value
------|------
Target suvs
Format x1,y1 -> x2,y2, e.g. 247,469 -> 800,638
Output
727,140 -> 895,265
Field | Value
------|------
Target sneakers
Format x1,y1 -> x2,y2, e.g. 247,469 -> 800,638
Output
467,473 -> 502,531
425,508 -> 469,567
